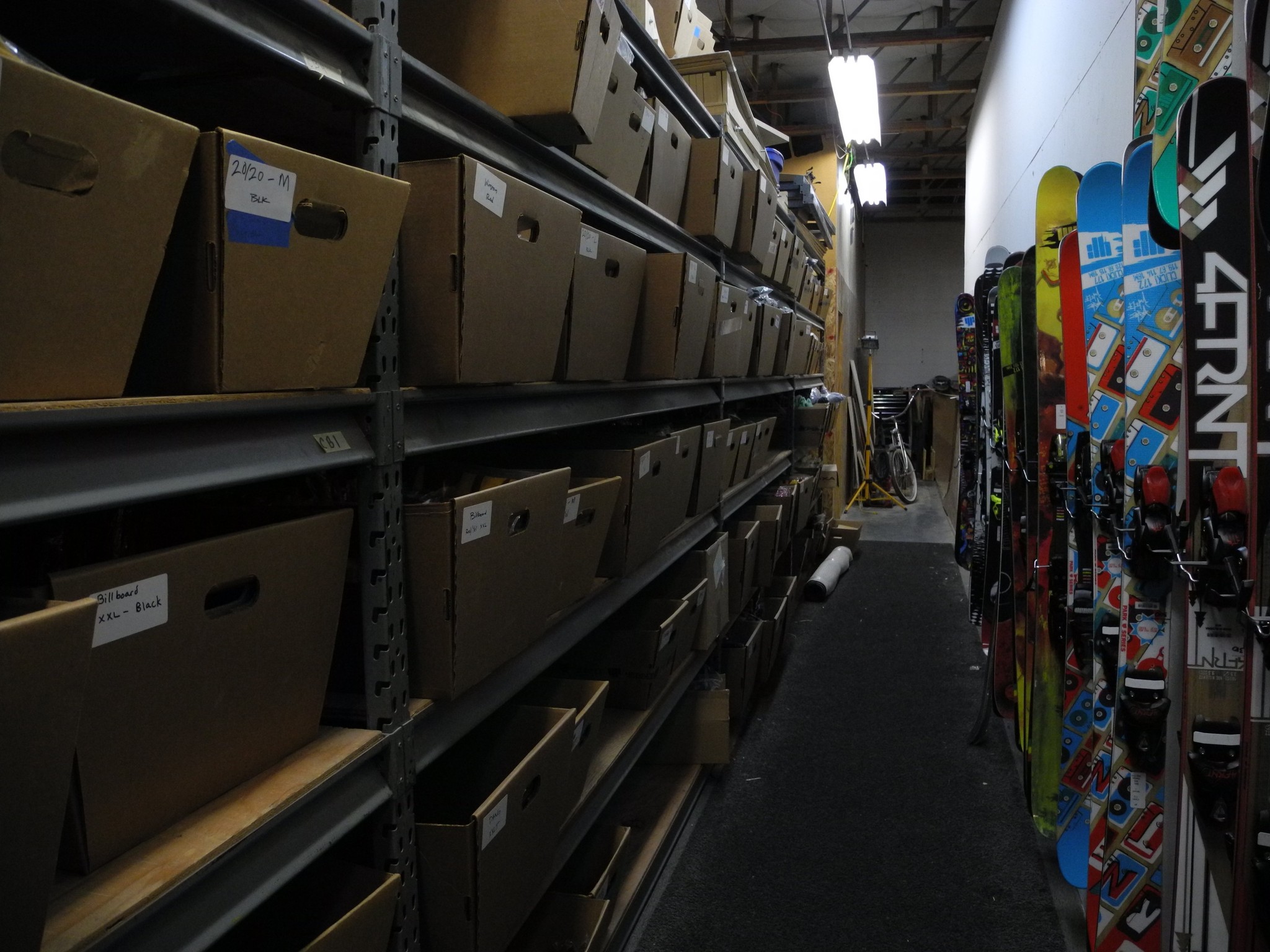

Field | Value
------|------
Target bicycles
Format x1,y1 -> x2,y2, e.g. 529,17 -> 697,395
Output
869,386 -> 922,503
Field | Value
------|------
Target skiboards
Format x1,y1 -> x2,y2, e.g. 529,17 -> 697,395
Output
952,0 -> 1269,951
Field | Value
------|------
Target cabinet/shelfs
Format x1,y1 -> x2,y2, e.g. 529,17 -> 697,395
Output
0,0 -> 836,952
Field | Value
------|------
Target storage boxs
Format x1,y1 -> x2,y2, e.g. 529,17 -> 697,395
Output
1,0 -> 829,952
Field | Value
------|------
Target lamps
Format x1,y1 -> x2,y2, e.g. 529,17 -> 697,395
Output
827,45 -> 883,150
854,158 -> 891,209
